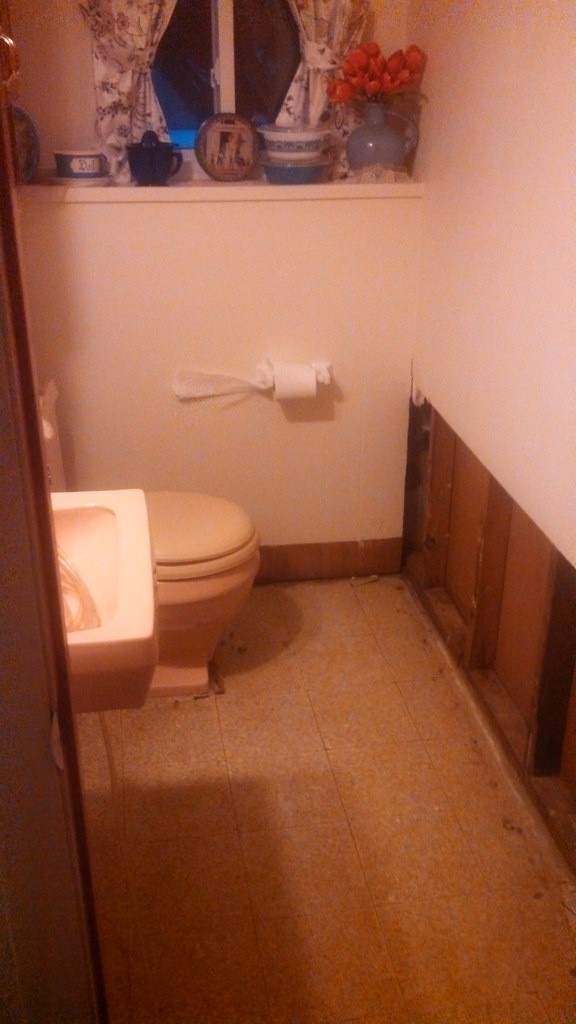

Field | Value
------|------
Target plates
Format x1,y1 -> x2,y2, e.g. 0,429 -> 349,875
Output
54,178 -> 111,187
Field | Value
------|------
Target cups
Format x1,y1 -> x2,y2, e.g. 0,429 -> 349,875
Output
124,142 -> 183,187
53,149 -> 108,178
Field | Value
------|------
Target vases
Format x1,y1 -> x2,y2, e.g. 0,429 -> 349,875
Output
344,105 -> 419,184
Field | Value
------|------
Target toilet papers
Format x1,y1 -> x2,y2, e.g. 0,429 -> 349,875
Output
272,364 -> 316,403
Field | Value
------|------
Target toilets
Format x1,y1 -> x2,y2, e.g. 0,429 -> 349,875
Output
34,376 -> 260,697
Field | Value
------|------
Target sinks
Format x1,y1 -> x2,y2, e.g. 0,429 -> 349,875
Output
48,488 -> 158,715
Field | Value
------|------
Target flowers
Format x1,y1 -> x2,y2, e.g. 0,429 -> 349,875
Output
324,38 -> 429,110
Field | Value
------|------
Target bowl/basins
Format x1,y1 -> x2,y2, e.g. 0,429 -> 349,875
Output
260,159 -> 330,184
255,122 -> 331,161
